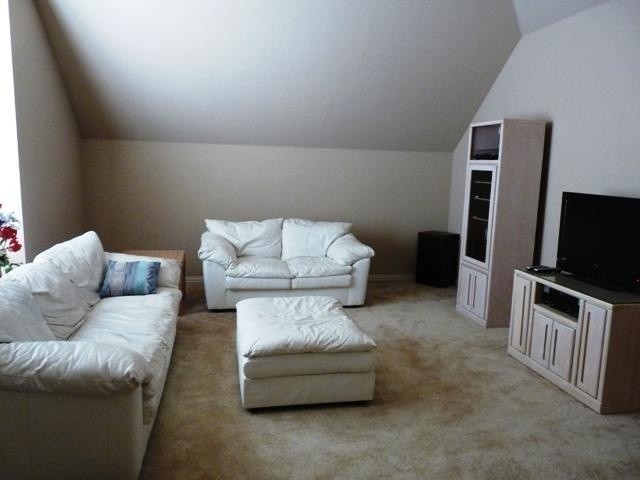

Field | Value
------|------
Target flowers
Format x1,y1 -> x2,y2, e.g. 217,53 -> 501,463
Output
0,212 -> 22,274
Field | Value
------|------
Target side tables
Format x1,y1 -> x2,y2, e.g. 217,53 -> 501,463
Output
124,250 -> 185,317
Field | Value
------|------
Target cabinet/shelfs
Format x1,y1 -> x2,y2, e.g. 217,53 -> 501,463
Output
508,267 -> 640,414
455,117 -> 552,329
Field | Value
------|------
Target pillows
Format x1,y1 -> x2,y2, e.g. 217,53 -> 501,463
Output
100,261 -> 161,297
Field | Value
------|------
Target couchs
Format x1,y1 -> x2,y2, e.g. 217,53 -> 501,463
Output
199,218 -> 375,309
0,230 -> 183,480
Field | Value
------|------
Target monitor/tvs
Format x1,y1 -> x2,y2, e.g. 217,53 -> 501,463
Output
556,190 -> 640,295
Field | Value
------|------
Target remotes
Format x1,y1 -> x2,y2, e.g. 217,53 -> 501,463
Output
534,267 -> 556,275
526,266 -> 550,272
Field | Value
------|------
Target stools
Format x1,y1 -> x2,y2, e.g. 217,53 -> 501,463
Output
236,296 -> 380,409
